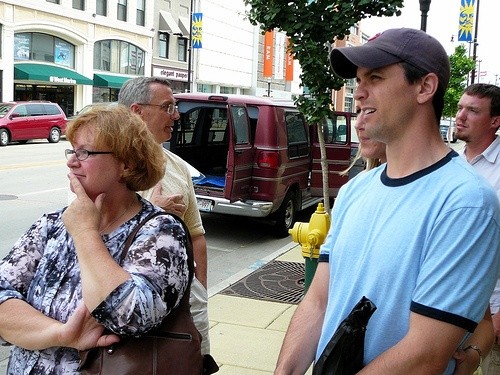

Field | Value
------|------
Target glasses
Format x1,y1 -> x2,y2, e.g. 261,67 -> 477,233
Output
137,103 -> 179,115
63,148 -> 115,162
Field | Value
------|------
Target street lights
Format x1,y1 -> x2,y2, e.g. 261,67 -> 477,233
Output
267,77 -> 272,98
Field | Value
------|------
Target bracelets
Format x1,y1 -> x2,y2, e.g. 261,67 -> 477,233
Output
463,345 -> 483,372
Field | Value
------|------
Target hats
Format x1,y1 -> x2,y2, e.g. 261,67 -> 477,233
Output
329,27 -> 450,93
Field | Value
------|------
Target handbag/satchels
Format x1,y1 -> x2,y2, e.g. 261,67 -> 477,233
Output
75,211 -> 219,375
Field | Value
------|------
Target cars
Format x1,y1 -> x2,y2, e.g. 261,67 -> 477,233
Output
0,101 -> 68,146
440,118 -> 457,142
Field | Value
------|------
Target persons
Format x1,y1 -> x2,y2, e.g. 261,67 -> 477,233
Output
274,28 -> 500,375
0,102 -> 192,375
118,78 -> 210,375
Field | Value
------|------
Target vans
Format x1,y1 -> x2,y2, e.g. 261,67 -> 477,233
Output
162,93 -> 382,240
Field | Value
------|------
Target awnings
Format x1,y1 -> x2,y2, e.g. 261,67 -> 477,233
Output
178,17 -> 190,35
14,62 -> 94,85
159,10 -> 182,33
94,74 -> 138,90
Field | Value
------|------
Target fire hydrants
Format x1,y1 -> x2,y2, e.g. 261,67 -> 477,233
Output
288,202 -> 332,296
